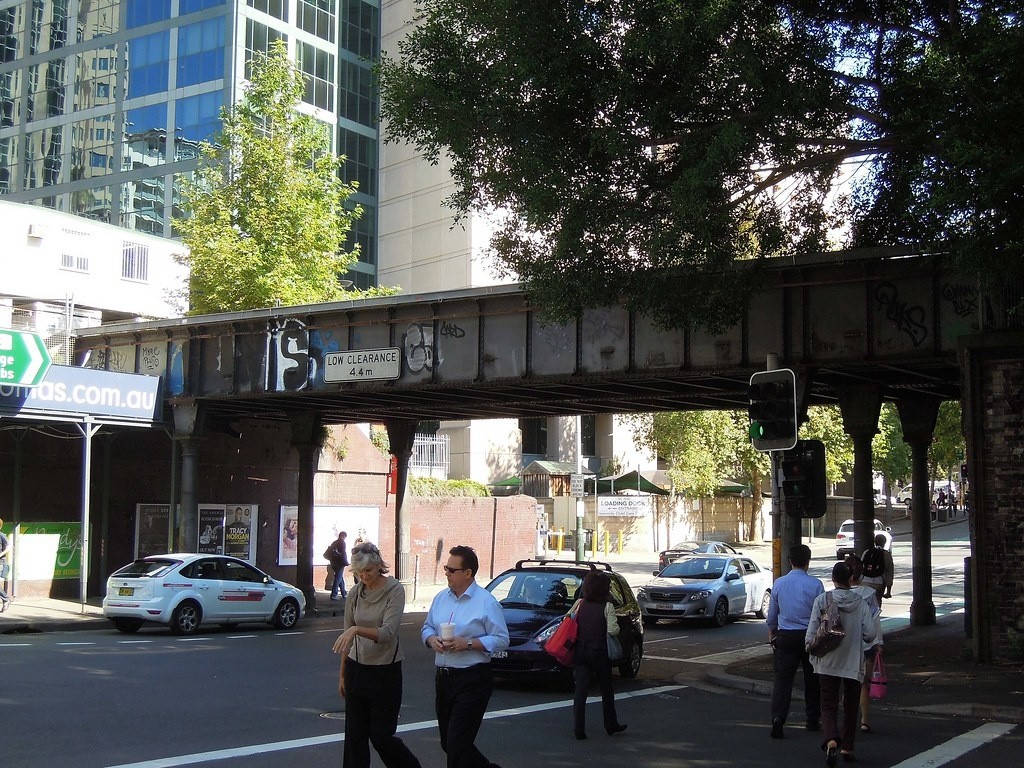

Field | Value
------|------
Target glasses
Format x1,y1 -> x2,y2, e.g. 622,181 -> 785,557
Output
444,566 -> 462,573
352,548 -> 377,555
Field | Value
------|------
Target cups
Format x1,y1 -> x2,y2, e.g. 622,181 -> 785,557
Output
440,623 -> 456,643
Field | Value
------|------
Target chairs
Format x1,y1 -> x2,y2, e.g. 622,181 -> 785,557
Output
228,567 -> 242,581
200,562 -> 215,578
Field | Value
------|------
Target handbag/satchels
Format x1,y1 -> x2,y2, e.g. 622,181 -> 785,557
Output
533,599 -> 583,665
806,591 -> 845,658
323,546 -> 342,561
325,569 -> 335,590
868,652 -> 889,699
607,636 -> 622,659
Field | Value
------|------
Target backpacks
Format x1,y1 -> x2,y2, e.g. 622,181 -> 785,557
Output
861,548 -> 885,577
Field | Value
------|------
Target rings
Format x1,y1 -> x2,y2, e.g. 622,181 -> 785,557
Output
451,646 -> 454,649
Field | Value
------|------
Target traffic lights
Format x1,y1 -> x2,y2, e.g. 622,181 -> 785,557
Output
782,440 -> 827,518
748,369 -> 797,451
961,463 -> 968,477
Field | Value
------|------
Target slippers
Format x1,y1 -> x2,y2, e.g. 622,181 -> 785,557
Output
840,752 -> 855,760
824,746 -> 838,767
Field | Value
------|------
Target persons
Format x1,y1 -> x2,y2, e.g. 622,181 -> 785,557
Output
421,545 -> 510,768
767,544 -> 826,740
909,504 -> 912,520
332,541 -> 421,768
931,500 -> 937,522
805,562 -> 877,767
567,572 -> 629,741
283,518 -> 298,551
938,488 -> 958,516
523,577 -> 545,604
0,518 -> 12,613
329,531 -> 349,600
845,556 -> 883,732
965,491 -> 968,510
861,534 -> 894,609
354,528 -> 369,584
226,506 -> 250,552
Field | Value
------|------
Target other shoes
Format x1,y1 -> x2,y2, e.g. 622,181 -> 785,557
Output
343,595 -> 347,598
575,733 -> 587,739
330,595 -> 341,600
606,723 -> 627,736
2,599 -> 11,611
806,721 -> 823,728
932,520 -> 935,522
770,717 -> 783,738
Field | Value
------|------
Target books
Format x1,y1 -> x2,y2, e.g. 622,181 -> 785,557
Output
870,608 -> 882,620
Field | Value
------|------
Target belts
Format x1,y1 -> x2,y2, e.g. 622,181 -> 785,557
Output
440,667 -> 449,676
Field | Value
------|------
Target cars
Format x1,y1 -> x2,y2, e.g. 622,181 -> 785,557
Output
658,540 -> 743,573
874,494 -> 896,506
635,552 -> 774,628
102,552 -> 307,636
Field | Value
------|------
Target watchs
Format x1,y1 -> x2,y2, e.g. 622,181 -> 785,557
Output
466,639 -> 473,650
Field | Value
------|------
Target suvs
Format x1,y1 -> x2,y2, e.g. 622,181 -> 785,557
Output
835,519 -> 893,560
483,558 -> 645,693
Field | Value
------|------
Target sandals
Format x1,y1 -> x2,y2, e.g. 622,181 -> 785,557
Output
860,723 -> 872,734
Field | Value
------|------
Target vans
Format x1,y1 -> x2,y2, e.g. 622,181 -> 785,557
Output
896,480 -> 957,505
